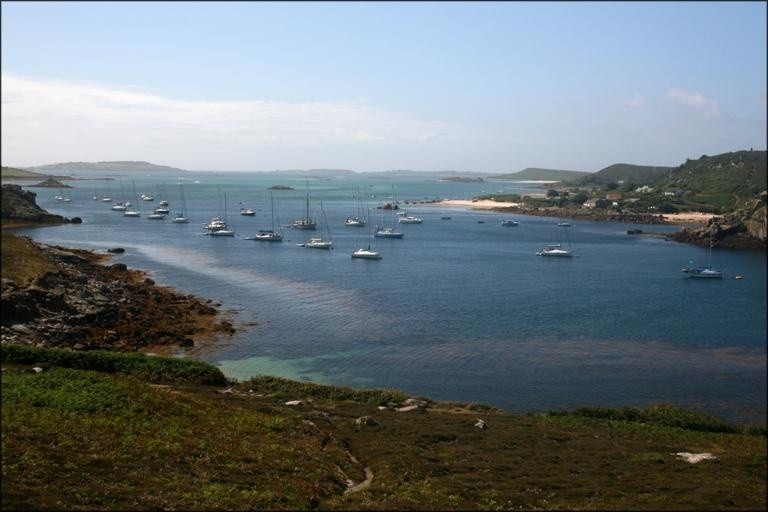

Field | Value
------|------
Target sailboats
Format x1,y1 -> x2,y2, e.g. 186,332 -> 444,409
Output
256,190 -> 284,242
303,198 -> 334,250
291,180 -> 317,230
680,229 -> 727,279
344,185 -> 367,227
372,190 -> 405,239
350,206 -> 384,261
50,175 -> 189,223
557,211 -> 574,226
534,211 -> 577,258
200,192 -> 235,236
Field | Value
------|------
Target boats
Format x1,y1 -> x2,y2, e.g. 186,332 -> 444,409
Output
397,215 -> 425,224
395,209 -> 407,215
736,274 -> 745,279
240,208 -> 257,216
500,218 -> 521,226
201,217 -> 233,230
